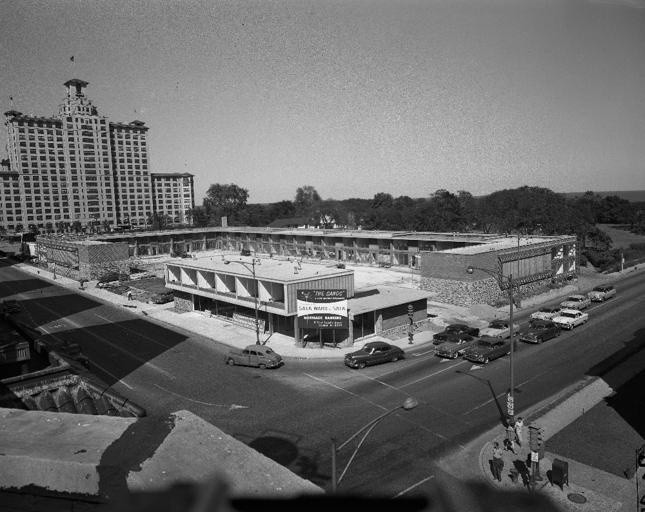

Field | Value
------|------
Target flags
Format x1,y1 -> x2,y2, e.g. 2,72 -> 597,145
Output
70,56 -> 74,63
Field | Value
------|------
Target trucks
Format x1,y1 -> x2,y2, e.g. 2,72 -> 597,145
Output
53,337 -> 89,369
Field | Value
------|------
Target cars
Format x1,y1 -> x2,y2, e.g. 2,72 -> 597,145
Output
226,346 -> 284,370
588,283 -> 615,303
517,295 -> 590,343
3,299 -> 22,314
432,321 -> 519,364
345,342 -> 404,369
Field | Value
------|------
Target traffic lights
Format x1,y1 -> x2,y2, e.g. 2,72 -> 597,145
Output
538,429 -> 543,452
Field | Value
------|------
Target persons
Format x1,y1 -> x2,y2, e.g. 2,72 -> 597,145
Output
492,442 -> 505,481
526,451 -> 543,485
127,289 -> 132,300
514,417 -> 526,449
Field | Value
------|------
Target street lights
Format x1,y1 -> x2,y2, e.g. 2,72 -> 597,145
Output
224,260 -> 259,346
467,266 -> 514,421
329,398 -> 418,494
